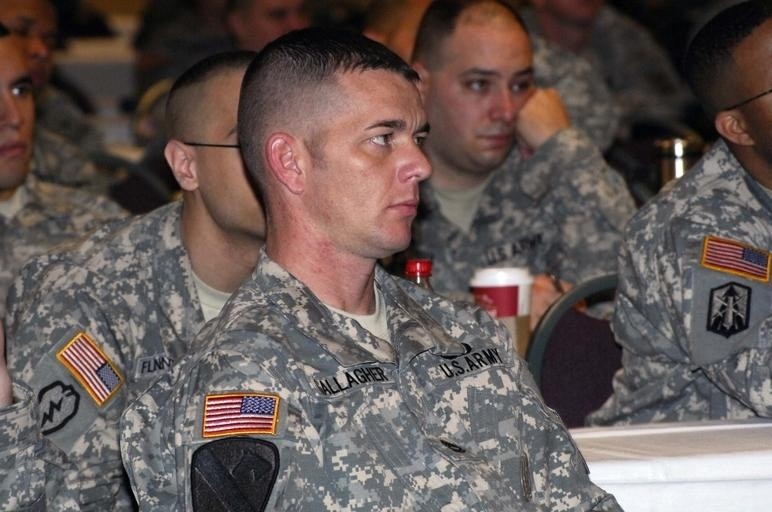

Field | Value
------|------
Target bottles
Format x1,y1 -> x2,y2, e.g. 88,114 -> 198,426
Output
405,257 -> 435,293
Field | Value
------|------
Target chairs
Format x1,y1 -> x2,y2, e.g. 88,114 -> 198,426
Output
525,273 -> 623,428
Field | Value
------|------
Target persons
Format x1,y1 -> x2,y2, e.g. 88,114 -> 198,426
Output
584,2 -> 772,424
0,0 -> 736,245
0,49 -> 261,512
382,0 -> 640,366
0,25 -> 131,322
175,25 -> 621,512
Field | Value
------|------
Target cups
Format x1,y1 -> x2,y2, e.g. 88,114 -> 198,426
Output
469,266 -> 532,357
647,132 -> 702,189
